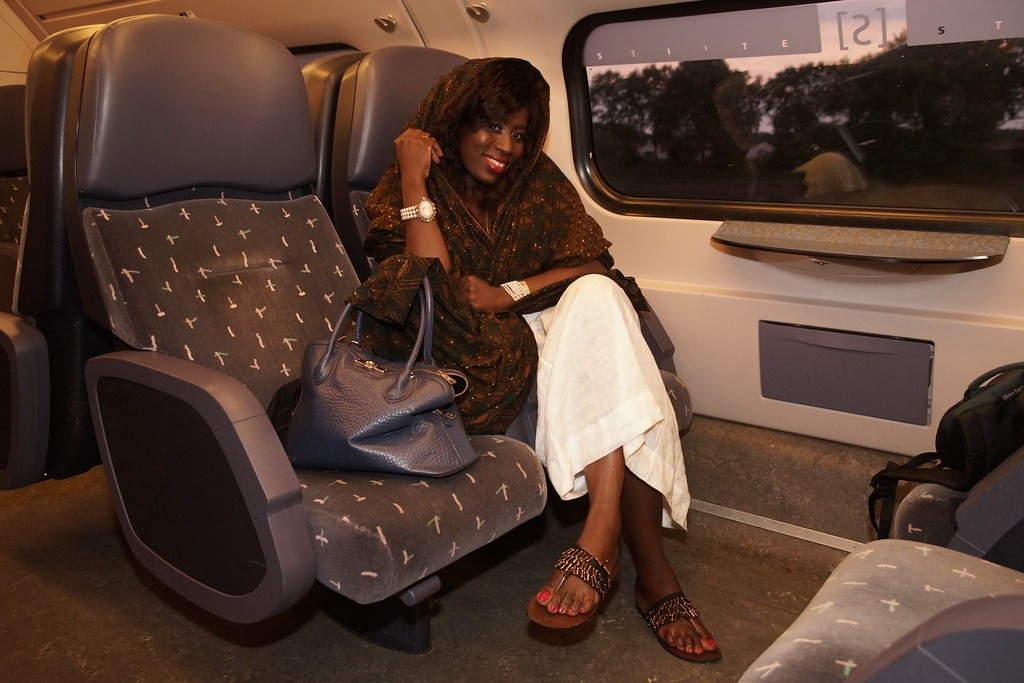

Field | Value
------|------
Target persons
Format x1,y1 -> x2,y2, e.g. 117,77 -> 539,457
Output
362,56 -> 721,665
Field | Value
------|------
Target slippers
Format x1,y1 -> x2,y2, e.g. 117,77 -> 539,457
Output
636,590 -> 724,663
520,544 -> 623,633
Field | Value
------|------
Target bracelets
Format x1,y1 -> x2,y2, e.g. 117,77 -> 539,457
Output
501,281 -> 530,300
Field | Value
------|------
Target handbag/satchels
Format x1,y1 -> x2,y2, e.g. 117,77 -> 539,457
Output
266,269 -> 480,480
935,360 -> 1023,482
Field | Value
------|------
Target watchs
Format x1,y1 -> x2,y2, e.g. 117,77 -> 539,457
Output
400,196 -> 437,222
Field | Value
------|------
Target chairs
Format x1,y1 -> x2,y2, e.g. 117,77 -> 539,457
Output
0,13 -> 1023,683
